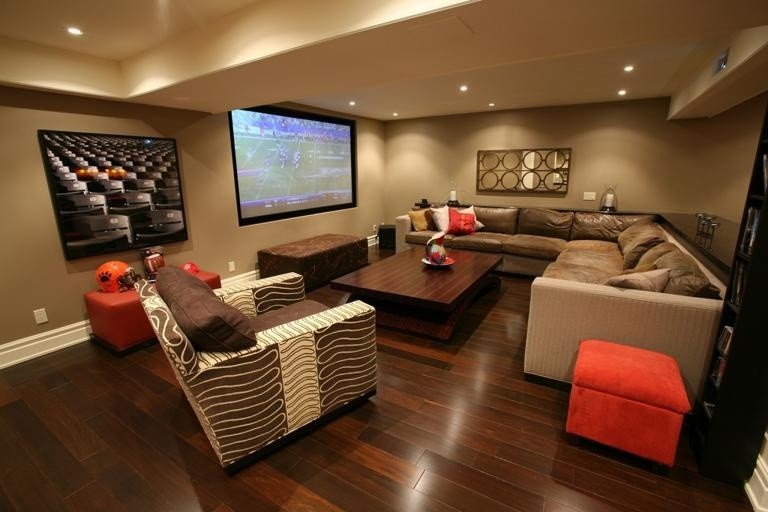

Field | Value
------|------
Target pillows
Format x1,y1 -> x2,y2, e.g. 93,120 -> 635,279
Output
409,205 -> 485,235
155,266 -> 258,351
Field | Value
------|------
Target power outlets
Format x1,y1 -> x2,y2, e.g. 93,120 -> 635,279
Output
33,308 -> 48,325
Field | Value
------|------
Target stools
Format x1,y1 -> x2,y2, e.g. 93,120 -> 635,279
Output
566,338 -> 694,469
85,269 -> 222,353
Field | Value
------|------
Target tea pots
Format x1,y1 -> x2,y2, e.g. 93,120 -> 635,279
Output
425,229 -> 448,263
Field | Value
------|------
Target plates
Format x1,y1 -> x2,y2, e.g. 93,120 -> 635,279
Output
420,256 -> 455,266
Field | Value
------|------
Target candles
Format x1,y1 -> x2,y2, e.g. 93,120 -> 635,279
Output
450,191 -> 456,200
604,194 -> 614,207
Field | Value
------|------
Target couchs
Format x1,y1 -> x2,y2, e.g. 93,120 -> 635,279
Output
396,204 -> 728,416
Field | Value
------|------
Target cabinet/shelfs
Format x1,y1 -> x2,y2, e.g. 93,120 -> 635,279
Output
682,93 -> 766,483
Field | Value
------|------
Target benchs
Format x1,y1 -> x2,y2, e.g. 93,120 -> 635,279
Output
257,235 -> 371,288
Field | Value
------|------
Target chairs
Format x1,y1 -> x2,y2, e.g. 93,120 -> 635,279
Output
134,271 -> 377,475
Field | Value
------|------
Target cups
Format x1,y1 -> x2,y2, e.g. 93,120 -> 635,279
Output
116,270 -> 138,289
